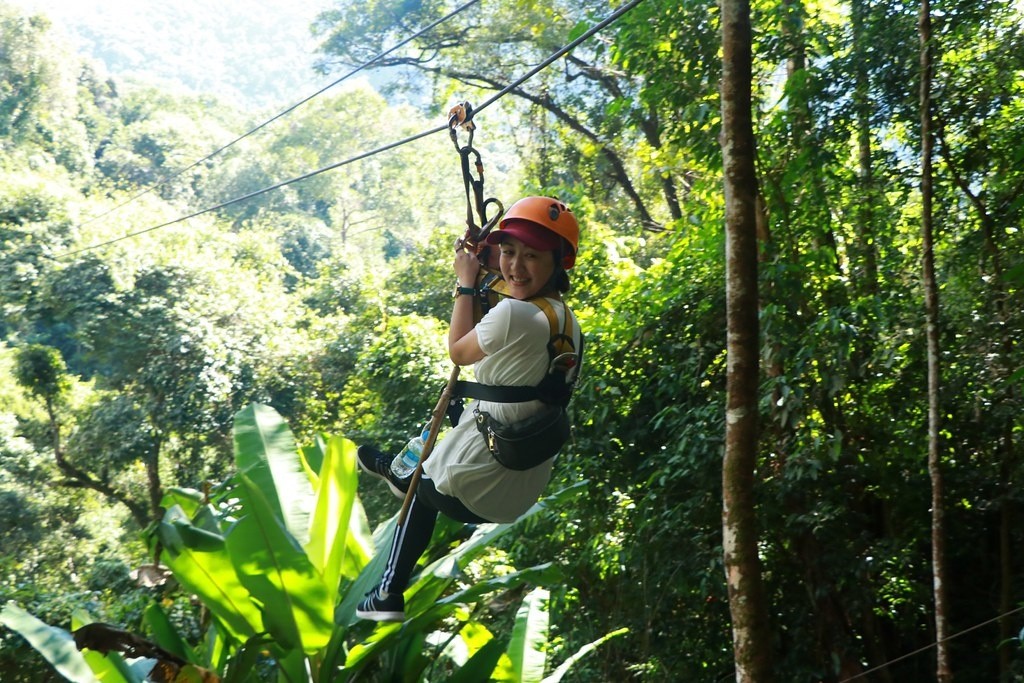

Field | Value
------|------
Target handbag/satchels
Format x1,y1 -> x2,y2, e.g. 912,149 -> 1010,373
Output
473,403 -> 571,471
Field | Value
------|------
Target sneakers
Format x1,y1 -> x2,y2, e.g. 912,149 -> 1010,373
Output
356,445 -> 412,500
356,585 -> 405,623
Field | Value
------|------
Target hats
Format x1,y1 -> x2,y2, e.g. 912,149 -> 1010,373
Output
485,220 -> 562,251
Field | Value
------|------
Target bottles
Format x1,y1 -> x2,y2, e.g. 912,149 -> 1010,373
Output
390,431 -> 430,478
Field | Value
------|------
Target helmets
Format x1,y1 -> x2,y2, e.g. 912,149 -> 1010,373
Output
499,196 -> 579,254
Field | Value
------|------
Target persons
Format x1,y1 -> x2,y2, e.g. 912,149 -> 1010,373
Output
355,196 -> 585,621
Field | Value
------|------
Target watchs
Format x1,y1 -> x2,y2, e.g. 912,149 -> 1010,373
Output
451,282 -> 475,298
